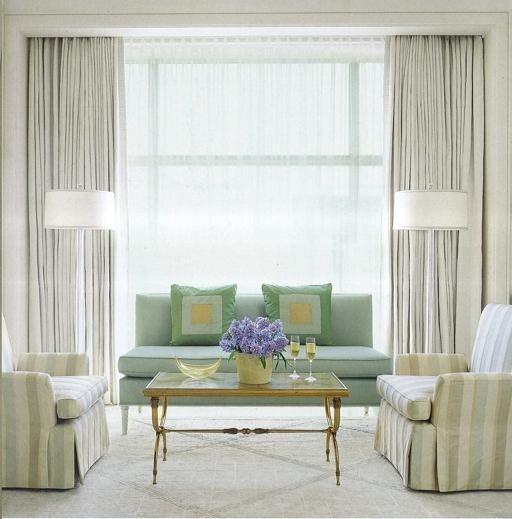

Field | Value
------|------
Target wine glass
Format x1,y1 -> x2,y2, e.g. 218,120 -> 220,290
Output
288,336 -> 316,381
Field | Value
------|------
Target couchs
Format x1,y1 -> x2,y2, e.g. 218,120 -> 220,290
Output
117,291 -> 392,436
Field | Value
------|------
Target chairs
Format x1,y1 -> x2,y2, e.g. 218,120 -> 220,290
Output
0,313 -> 110,490
374,303 -> 512,492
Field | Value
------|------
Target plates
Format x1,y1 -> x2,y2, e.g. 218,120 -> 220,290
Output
175,356 -> 222,379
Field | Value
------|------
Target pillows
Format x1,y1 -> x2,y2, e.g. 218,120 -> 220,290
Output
261,282 -> 333,346
170,283 -> 238,346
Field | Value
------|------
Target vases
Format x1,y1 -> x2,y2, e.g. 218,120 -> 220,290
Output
236,354 -> 272,385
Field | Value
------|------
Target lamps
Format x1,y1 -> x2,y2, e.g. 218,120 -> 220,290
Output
43,189 -> 117,353
393,190 -> 468,356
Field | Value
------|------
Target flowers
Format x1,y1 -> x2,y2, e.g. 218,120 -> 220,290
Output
218,316 -> 290,371
